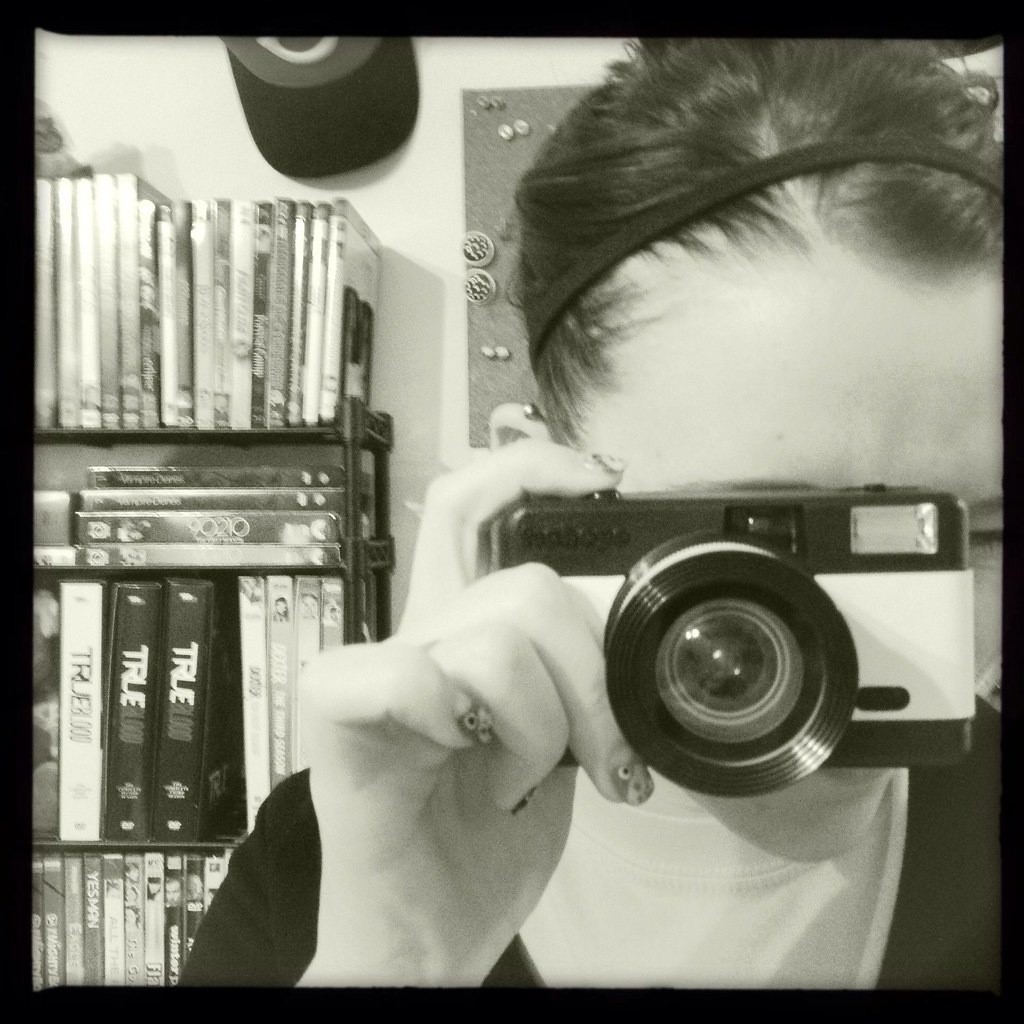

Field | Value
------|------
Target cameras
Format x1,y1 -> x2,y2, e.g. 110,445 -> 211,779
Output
485,487 -> 977,797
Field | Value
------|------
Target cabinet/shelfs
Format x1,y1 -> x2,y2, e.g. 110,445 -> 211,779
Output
32,397 -> 396,983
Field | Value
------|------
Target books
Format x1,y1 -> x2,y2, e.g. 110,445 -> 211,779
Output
35,173 -> 381,428
27,457 -> 382,989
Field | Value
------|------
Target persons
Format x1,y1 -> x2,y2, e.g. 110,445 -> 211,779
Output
174,32 -> 1003,988
299,593 -> 319,618
272,598 -> 290,622
